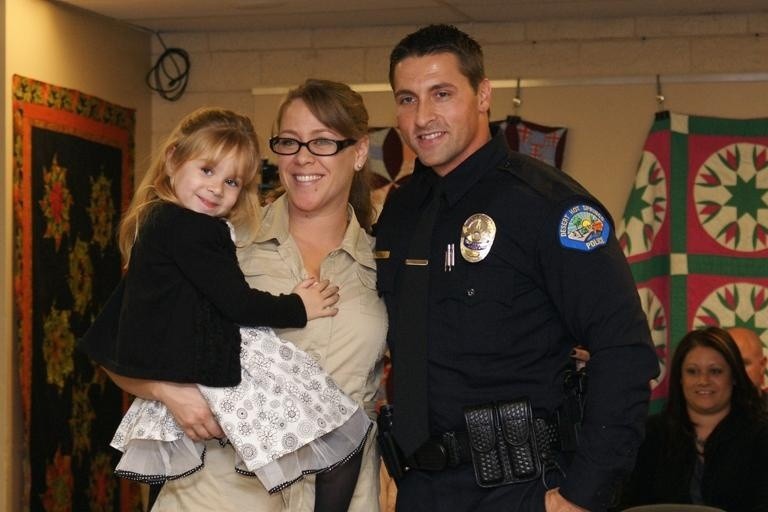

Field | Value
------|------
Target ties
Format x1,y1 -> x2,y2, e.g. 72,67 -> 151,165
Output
391,179 -> 442,456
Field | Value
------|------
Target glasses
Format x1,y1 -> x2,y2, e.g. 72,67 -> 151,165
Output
270,135 -> 357,156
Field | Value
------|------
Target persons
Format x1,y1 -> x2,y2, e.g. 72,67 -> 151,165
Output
730,328 -> 768,404
370,24 -> 661,512
621,326 -> 768,512
79,108 -> 373,494
148,77 -> 391,512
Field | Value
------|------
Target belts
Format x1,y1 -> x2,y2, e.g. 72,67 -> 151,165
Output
407,422 -> 560,469
